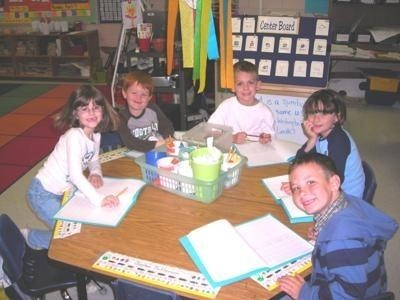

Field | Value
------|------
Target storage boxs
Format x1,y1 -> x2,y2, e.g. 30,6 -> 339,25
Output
330,72 -> 366,98
366,92 -> 397,106
64,44 -> 87,56
332,22 -> 400,51
366,69 -> 400,92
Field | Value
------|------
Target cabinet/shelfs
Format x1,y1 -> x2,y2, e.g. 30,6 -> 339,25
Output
0,29 -> 100,81
130,12 -> 206,128
329,1 -> 400,63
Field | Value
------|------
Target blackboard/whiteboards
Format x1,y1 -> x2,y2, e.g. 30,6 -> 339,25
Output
254,90 -> 318,146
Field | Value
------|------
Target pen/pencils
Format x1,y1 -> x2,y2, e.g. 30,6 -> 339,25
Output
115,187 -> 128,200
227,149 -> 238,162
247,135 -> 259,137
226,146 -> 233,162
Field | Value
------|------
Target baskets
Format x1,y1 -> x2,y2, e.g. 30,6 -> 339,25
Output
134,138 -> 248,204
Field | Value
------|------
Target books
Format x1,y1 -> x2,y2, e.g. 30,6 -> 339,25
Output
260,173 -> 315,223
232,140 -> 302,168
56,38 -> 61,56
53,175 -> 146,229
331,43 -> 400,62
32,21 -> 69,33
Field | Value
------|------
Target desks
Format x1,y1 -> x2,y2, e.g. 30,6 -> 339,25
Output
46,128 -> 318,300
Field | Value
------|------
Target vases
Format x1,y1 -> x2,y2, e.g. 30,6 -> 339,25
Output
150,38 -> 165,52
136,38 -> 152,52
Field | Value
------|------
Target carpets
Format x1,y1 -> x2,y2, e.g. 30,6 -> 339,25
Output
0,75 -> 173,194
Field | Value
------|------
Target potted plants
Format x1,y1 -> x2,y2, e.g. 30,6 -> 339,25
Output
91,56 -> 107,83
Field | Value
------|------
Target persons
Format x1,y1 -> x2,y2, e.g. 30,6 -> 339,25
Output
204,60 -> 280,146
278,154 -> 397,300
16,84 -> 120,248
115,70 -> 178,152
179,214 -> 316,285
292,88 -> 366,202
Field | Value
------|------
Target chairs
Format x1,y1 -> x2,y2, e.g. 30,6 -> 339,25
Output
361,160 -> 377,203
367,290 -> 395,300
0,212 -> 101,300
91,132 -> 125,155
140,69 -> 188,130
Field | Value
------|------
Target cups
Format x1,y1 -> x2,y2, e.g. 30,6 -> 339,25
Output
157,157 -> 181,190
177,160 -> 195,194
189,147 -> 224,204
167,141 -> 188,156
31,20 -> 68,36
221,153 -> 242,188
146,151 -> 167,181
180,146 -> 197,160
